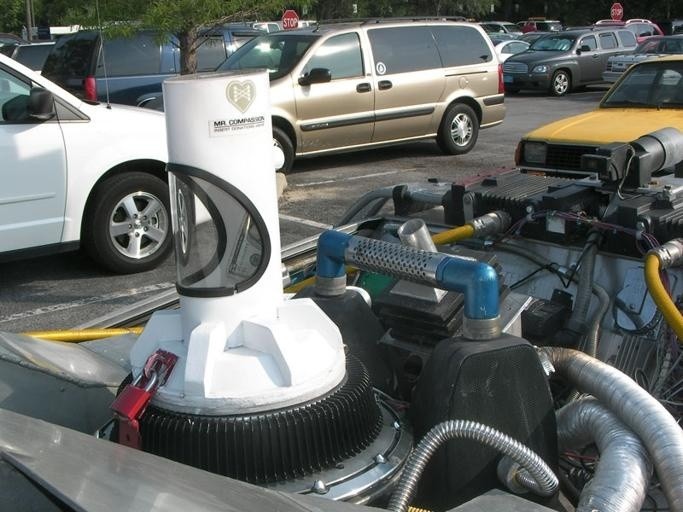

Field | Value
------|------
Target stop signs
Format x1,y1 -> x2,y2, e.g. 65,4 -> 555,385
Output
281,8 -> 299,29
610,3 -> 623,20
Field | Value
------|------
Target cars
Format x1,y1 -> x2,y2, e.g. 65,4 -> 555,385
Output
466,15 -> 682,178
